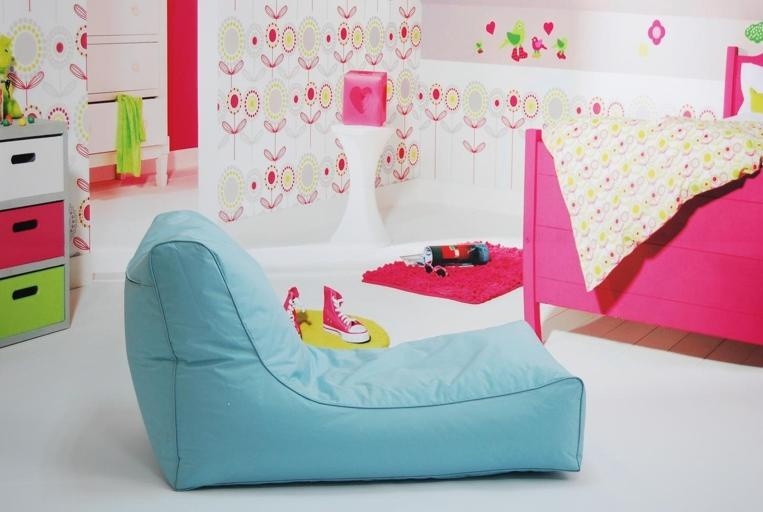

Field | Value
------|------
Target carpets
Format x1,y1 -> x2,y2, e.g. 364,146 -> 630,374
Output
361,241 -> 528,305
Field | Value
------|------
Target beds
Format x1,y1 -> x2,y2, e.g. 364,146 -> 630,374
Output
524,43 -> 763,347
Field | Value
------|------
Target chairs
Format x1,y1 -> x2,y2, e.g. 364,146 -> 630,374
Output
124,211 -> 584,491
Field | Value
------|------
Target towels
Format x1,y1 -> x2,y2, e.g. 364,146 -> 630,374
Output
116,94 -> 145,178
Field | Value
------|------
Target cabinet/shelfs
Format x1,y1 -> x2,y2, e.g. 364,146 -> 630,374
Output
86,0 -> 170,188
0,121 -> 71,347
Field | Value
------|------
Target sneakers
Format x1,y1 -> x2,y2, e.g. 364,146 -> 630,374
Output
321,285 -> 372,346
